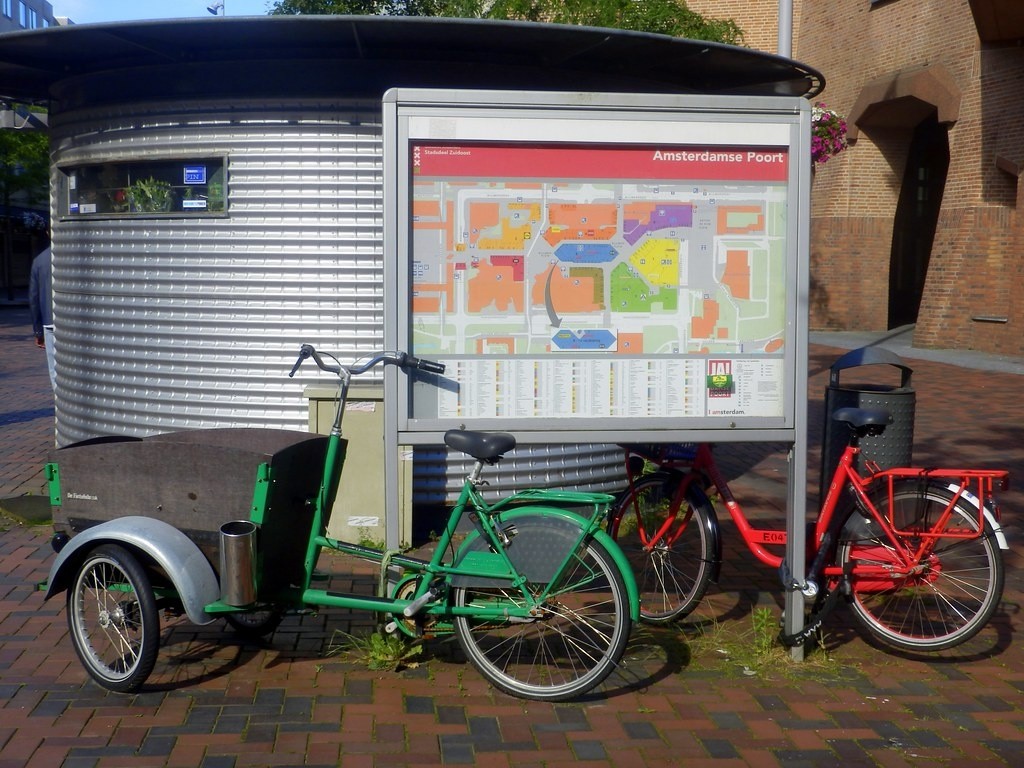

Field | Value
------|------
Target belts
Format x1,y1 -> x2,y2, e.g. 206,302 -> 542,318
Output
46,328 -> 53,330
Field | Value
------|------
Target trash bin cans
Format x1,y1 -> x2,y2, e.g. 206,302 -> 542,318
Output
819,347 -> 916,565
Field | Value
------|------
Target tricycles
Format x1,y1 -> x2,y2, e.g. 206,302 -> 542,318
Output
43,342 -> 643,702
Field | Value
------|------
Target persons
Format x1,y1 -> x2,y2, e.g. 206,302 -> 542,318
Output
84,182 -> 113,213
28,219 -> 55,399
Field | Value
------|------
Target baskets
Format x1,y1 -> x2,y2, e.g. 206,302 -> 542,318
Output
632,442 -> 697,461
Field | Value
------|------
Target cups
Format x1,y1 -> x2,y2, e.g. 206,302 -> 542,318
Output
219,519 -> 258,608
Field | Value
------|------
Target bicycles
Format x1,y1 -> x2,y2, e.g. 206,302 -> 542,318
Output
603,405 -> 1011,652
